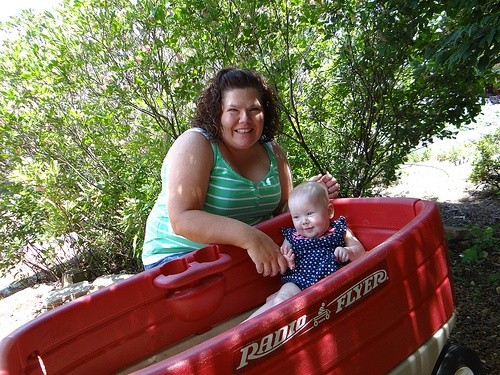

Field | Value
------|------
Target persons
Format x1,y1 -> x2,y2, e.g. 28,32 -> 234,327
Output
238,181 -> 365,325
141,68 -> 343,277
